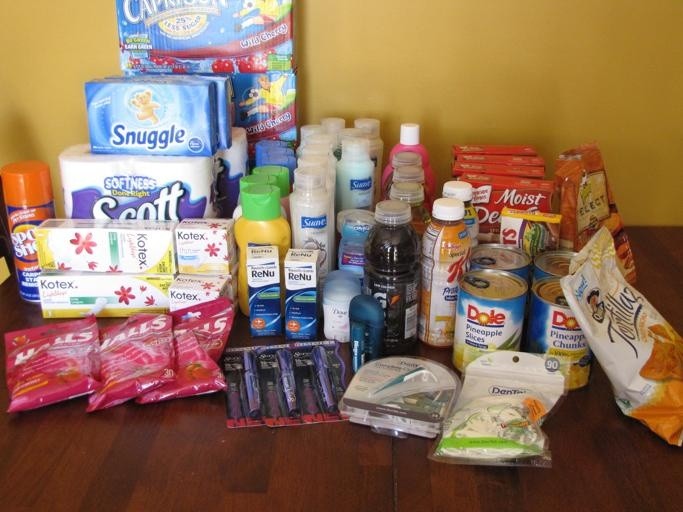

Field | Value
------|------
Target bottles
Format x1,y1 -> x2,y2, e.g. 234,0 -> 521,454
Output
0,159 -> 56,304
233,117 -> 479,376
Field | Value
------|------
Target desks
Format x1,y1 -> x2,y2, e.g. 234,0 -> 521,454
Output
0,225 -> 683,511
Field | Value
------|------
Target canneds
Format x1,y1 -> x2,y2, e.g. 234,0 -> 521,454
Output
452,241 -> 591,391
